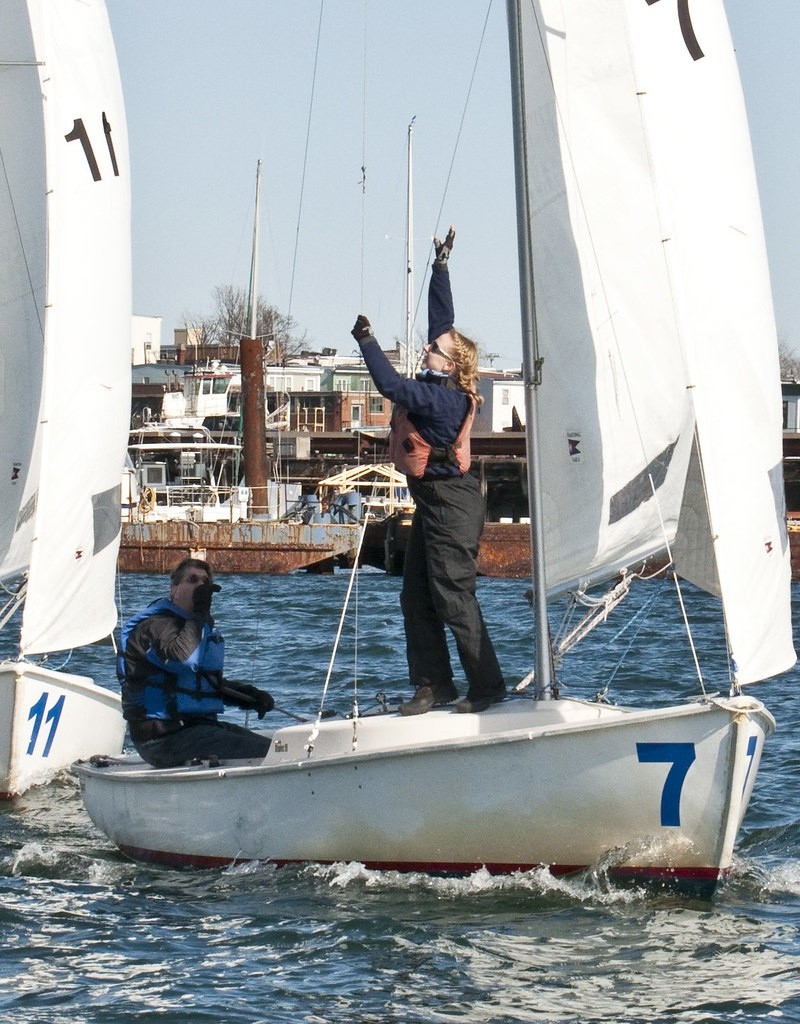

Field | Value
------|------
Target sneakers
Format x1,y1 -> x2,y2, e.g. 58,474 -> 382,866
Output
457,686 -> 509,713
398,680 -> 458,715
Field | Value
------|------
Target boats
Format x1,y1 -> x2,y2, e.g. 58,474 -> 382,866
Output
118,517 -> 365,575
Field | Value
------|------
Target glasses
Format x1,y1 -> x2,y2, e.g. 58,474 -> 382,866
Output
431,342 -> 455,360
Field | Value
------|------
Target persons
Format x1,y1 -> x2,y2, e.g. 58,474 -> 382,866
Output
351,227 -> 506,717
117,558 -> 275,767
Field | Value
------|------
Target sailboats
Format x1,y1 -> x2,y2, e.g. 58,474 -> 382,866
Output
1,0 -> 129,799
72,0 -> 800,897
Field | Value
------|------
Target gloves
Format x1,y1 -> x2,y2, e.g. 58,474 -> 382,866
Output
350,314 -> 373,339
233,684 -> 276,719
433,227 -> 456,266
193,582 -> 221,621
